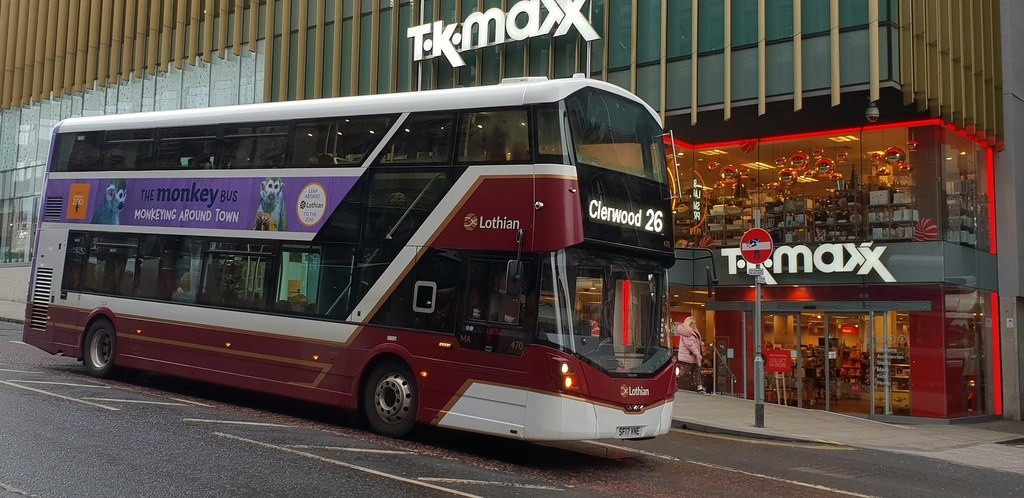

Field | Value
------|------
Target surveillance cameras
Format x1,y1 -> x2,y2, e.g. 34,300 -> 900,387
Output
867,107 -> 879,122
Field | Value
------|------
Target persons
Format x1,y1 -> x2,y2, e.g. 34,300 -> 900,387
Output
484,264 -> 550,342
67,261 -> 309,316
676,316 -> 707,394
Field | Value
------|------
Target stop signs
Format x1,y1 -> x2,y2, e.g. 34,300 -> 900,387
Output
738,228 -> 774,265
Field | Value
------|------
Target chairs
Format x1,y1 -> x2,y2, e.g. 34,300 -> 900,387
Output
84,139 -> 543,349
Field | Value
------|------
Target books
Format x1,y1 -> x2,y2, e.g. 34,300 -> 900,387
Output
867,189 -> 919,240
945,179 -> 977,245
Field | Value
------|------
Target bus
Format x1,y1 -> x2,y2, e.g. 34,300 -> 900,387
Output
19,71 -> 678,443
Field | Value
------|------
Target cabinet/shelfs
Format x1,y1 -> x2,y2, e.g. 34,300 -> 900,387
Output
675,183 -> 976,245
759,342 -> 908,404
